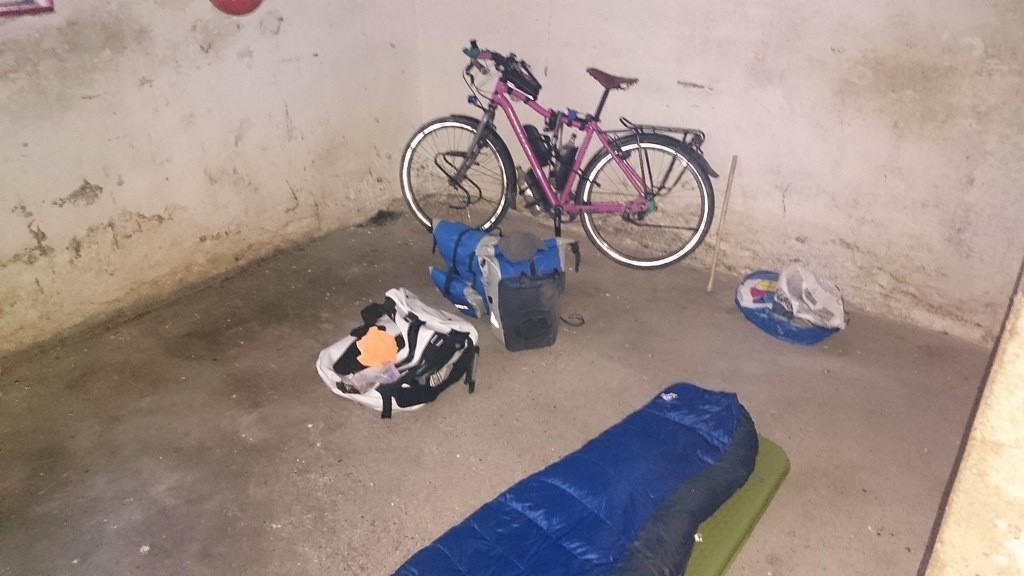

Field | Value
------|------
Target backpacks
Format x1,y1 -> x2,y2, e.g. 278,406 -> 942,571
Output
315,288 -> 478,418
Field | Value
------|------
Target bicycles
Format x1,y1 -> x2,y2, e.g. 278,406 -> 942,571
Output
398,39 -> 720,270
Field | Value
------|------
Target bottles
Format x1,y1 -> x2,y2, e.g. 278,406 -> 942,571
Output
515,165 -> 546,217
548,132 -> 577,191
